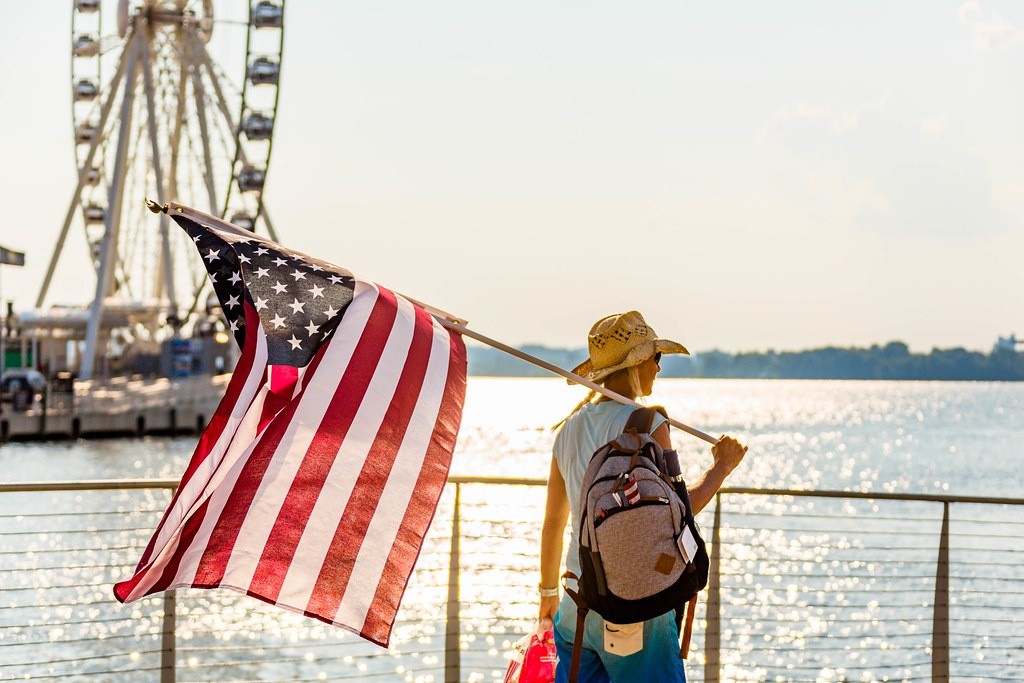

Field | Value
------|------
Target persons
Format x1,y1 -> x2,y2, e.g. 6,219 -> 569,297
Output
538,310 -> 748,683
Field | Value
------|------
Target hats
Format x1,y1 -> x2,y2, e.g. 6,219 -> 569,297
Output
566,310 -> 690,385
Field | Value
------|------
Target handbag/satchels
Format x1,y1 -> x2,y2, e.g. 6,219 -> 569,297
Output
504,617 -> 560,683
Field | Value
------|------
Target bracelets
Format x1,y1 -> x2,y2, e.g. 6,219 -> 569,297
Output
538,584 -> 560,597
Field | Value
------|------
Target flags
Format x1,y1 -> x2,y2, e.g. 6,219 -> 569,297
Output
113,212 -> 468,648
1,247 -> 25,267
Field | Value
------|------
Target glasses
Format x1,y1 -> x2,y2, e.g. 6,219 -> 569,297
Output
654,351 -> 661,364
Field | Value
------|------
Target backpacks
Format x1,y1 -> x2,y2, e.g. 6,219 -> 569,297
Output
561,405 -> 709,683
